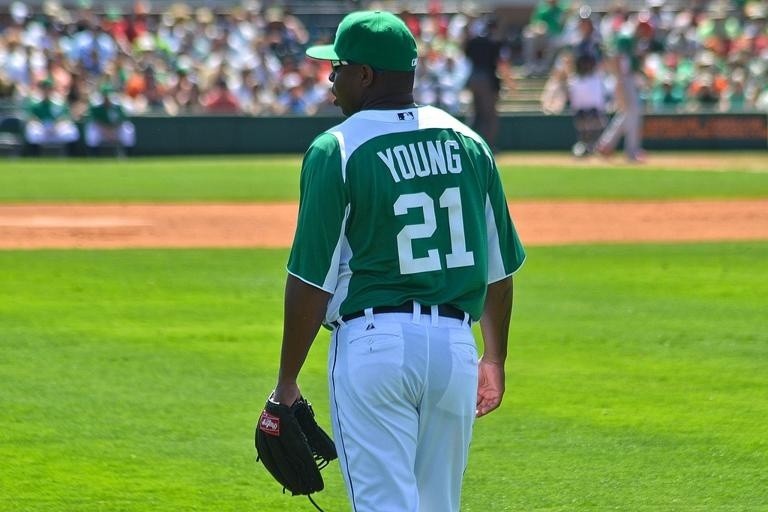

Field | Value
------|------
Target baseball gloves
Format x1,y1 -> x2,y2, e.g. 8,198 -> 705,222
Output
255,389 -> 337,511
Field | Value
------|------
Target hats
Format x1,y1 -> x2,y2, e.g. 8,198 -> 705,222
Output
306,9 -> 420,73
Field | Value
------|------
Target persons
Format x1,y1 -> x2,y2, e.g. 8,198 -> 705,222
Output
552,13 -> 610,153
254,8 -> 525,512
520,0 -> 768,114
595,22 -> 653,161
380,1 -> 510,143
2,1 -> 330,149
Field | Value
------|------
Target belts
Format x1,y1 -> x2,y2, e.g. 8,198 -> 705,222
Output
330,304 -> 471,328
